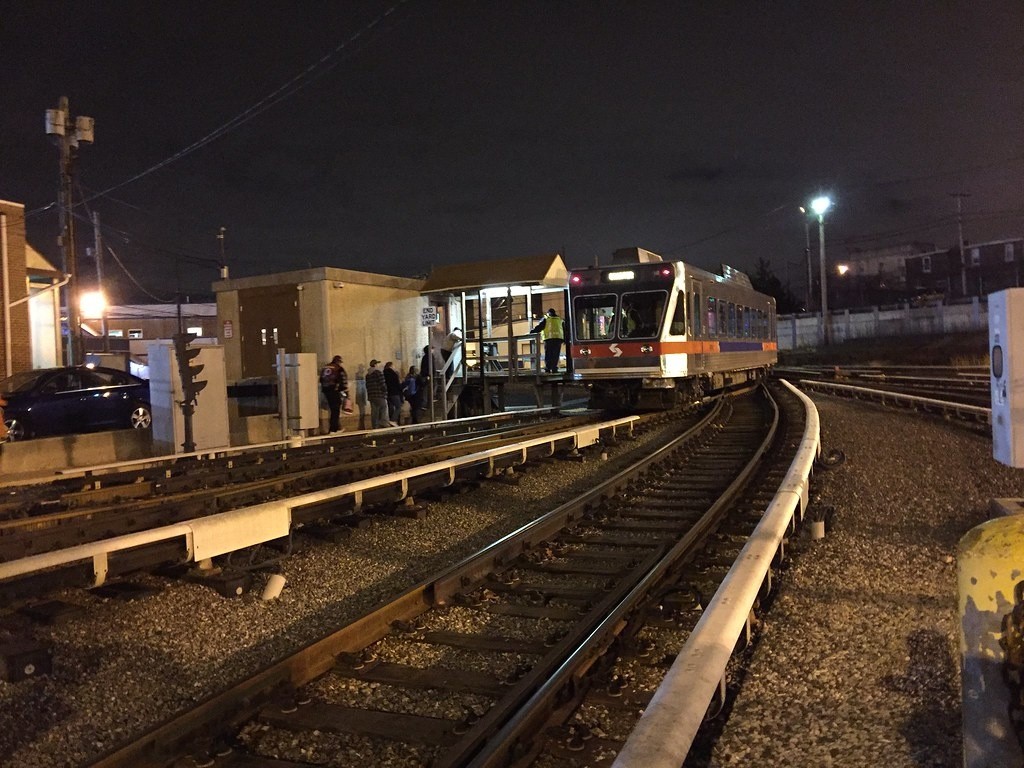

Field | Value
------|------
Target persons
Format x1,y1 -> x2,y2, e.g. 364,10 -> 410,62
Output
528,309 -> 567,373
420,346 -> 438,411
365,358 -> 390,429
405,365 -> 424,424
383,362 -> 403,427
440,327 -> 463,383
608,305 -> 628,340
623,301 -> 644,338
320,355 -> 350,435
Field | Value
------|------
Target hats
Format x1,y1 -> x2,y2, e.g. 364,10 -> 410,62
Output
369,359 -> 381,365
332,355 -> 343,363
424,344 -> 436,353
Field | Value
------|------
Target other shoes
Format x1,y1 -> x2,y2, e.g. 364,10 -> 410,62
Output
329,431 -> 340,435
389,420 -> 400,427
337,427 -> 346,434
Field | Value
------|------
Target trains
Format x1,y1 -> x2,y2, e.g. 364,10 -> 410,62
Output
565,248 -> 779,405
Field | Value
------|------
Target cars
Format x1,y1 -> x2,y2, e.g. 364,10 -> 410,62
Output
0,365 -> 152,444
